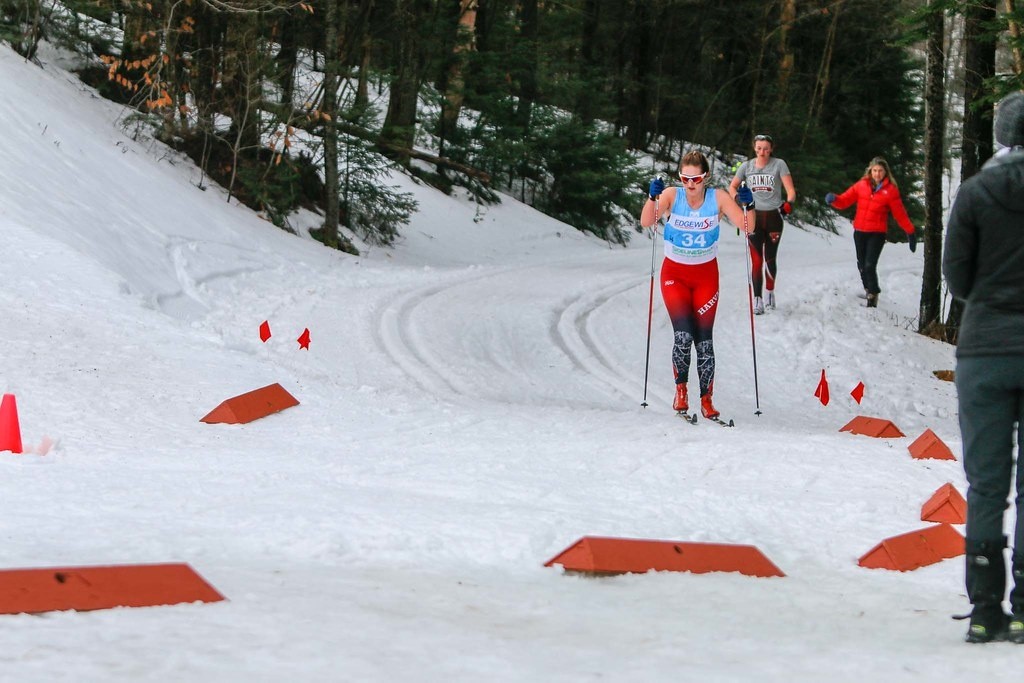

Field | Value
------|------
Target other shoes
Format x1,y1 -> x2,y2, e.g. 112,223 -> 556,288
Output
867,294 -> 878,307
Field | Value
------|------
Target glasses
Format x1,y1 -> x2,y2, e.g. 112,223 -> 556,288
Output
679,172 -> 706,184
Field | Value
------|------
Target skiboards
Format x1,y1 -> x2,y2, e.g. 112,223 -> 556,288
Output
677,410 -> 735,427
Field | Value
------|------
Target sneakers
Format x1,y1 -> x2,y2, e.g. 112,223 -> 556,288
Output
701,395 -> 720,417
967,605 -> 1011,642
673,385 -> 689,410
764,291 -> 775,309
753,296 -> 764,314
1008,613 -> 1024,643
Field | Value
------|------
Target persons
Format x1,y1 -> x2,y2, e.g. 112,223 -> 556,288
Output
943,91 -> 1024,644
641,151 -> 756,417
825,156 -> 916,308
728,135 -> 796,315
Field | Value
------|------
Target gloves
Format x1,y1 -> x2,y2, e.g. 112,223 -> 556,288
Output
826,194 -> 835,203
779,202 -> 791,216
908,234 -> 916,252
649,177 -> 665,201
737,186 -> 753,204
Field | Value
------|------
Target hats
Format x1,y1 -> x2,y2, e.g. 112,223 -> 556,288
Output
995,92 -> 1024,148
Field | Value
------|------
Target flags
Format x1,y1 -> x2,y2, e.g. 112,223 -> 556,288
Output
813,370 -> 829,405
851,381 -> 865,404
260,321 -> 271,342
0,394 -> 23,454
297,328 -> 310,350
